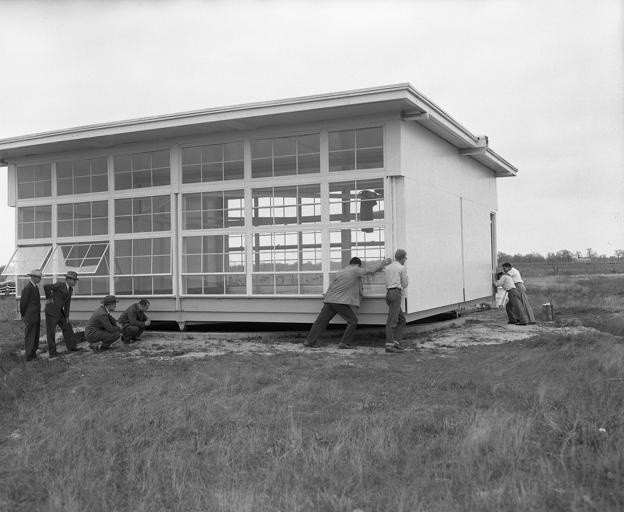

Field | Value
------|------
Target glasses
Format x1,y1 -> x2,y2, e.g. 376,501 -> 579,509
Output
403,256 -> 409,262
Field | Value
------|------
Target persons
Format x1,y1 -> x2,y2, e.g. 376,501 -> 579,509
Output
385,250 -> 408,353
118,300 -> 152,343
304,256 -> 392,349
43,271 -> 83,358
494,272 -> 529,325
20,269 -> 41,361
85,296 -> 124,353
503,263 -> 536,324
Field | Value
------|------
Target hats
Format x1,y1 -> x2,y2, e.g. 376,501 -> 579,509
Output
101,293 -> 119,305
30,267 -> 43,278
65,270 -> 78,281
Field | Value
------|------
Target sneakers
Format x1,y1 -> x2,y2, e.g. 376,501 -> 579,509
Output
338,341 -> 355,351
48,350 -> 66,359
24,356 -> 44,363
515,321 -> 529,327
301,338 -> 323,350
88,344 -> 115,353
507,320 -> 519,324
121,335 -> 132,345
66,344 -> 84,353
384,342 -> 405,354
528,320 -> 537,325
132,336 -> 143,342
